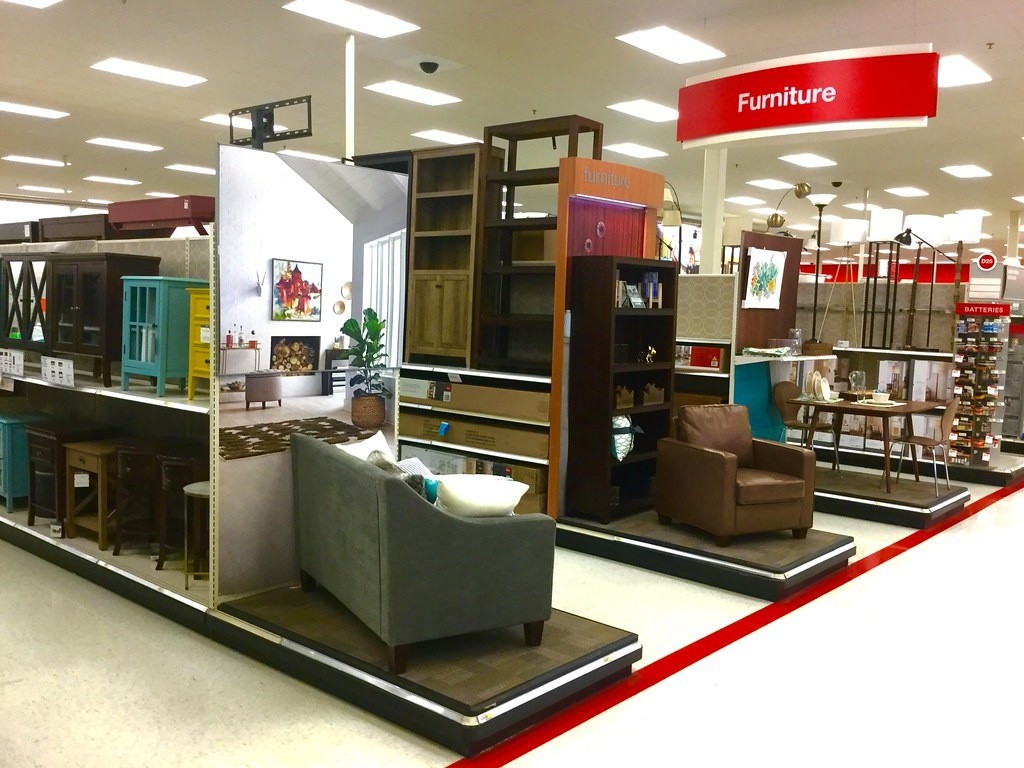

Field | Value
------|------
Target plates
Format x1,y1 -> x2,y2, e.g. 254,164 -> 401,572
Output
805,370 -> 830,401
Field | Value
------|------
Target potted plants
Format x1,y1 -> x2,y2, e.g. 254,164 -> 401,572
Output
335,337 -> 341,350
340,307 -> 393,427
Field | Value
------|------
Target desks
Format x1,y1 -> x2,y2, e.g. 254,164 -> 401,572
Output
65,442 -> 139,550
26,421 -> 123,529
220,343 -> 262,375
805,396 -> 940,494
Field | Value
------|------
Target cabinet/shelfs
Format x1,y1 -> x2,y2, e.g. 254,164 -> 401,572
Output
44,254 -> 161,388
0,233 -> 211,420
403,144 -> 504,367
0,253 -> 51,354
473,115 -> 605,370
573,256 -> 684,523
120,276 -> 209,400
0,413 -> 60,513
187,285 -> 214,398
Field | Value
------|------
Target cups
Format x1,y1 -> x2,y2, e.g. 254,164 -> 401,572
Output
789,328 -> 802,352
856,385 -> 866,404
848,370 -> 866,392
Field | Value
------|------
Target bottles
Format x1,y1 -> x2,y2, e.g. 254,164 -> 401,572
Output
232,324 -> 238,344
226,330 -> 234,348
248,330 -> 257,348
238,326 -> 245,347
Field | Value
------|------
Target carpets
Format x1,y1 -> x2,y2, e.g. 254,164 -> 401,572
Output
219,414 -> 373,462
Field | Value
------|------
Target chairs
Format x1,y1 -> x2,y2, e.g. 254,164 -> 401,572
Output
772,379 -> 839,473
878,399 -> 961,498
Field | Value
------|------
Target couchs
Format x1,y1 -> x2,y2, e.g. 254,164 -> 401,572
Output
656,402 -> 815,547
287,430 -> 556,672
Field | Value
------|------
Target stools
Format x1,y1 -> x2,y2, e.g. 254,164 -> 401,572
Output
246,372 -> 284,409
183,481 -> 211,590
152,438 -> 210,575
112,439 -> 163,555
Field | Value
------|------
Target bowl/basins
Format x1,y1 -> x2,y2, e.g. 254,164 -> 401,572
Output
830,392 -> 839,400
872,393 -> 890,402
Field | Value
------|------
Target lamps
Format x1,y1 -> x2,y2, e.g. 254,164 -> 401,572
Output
662,181 -> 683,273
802,194 -> 983,351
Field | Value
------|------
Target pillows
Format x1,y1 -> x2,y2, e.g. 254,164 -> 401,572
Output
330,432 -> 529,519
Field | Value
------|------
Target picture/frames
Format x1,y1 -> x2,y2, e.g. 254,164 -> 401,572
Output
272,258 -> 324,323
